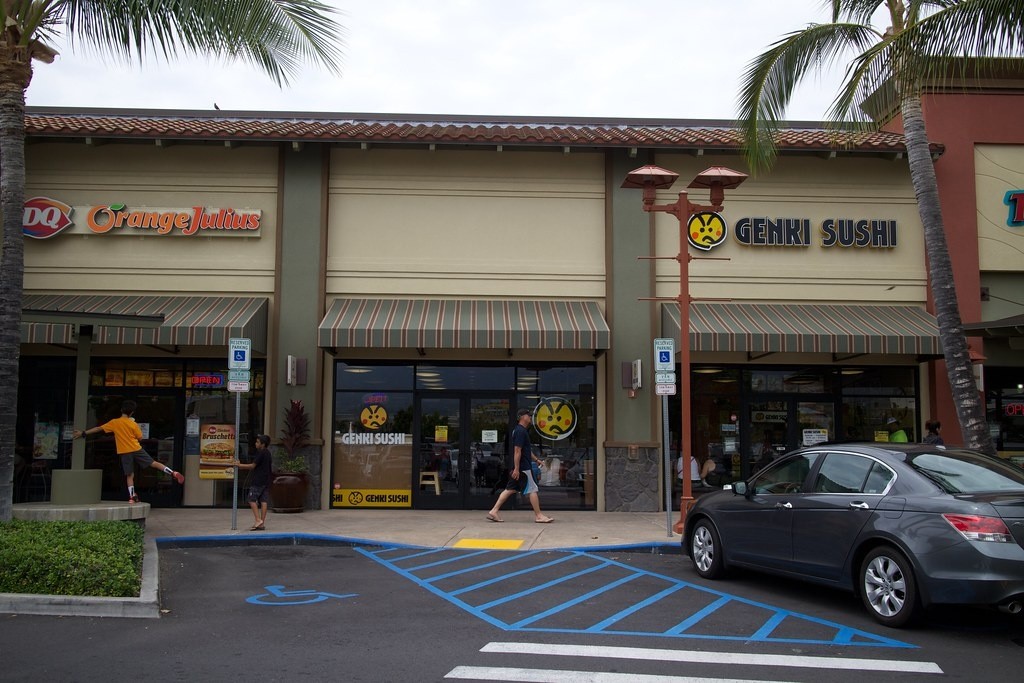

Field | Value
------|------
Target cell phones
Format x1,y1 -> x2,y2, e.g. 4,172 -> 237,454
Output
509,470 -> 520,478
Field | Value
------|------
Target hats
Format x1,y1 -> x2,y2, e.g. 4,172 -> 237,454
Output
517,408 -> 530,418
887,417 -> 897,424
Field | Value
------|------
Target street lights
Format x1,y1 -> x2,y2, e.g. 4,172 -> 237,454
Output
622,165 -> 750,536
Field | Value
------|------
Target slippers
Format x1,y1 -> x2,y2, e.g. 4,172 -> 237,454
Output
250,525 -> 265,531
486,517 -> 504,522
535,518 -> 554,523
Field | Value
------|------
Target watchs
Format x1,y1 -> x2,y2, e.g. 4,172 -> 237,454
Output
82,431 -> 86,438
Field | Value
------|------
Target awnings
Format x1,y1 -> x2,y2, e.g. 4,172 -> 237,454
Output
659,298 -> 952,360
316,297 -> 610,359
14,291 -> 272,357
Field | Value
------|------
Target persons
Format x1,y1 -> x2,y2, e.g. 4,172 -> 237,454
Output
676,448 -> 702,487
72,399 -> 185,503
699,451 -> 732,487
486,408 -> 555,523
886,416 -> 909,442
923,419 -> 945,446
233,434 -> 273,531
473,444 -> 484,488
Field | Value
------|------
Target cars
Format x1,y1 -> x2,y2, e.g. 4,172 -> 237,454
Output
680,446 -> 1024,629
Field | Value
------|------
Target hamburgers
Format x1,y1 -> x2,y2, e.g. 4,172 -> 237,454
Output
202,442 -> 234,463
158,454 -> 169,462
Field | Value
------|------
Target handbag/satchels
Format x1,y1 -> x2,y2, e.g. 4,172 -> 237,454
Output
506,470 -> 528,492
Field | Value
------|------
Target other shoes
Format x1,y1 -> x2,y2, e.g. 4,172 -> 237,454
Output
129,493 -> 138,504
173,471 -> 184,483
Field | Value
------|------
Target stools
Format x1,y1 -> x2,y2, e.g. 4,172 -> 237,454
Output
420,472 -> 441,495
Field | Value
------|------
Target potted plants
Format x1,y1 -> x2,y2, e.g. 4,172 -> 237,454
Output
270,400 -> 311,513
